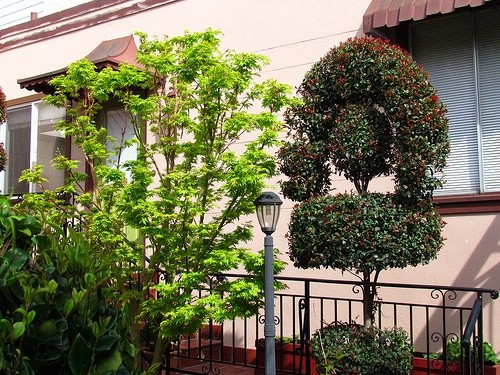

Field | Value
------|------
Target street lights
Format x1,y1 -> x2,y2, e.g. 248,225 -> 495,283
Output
251,192 -> 284,375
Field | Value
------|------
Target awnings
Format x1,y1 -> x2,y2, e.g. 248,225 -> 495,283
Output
362,0 -> 487,34
18,34 -> 162,114
